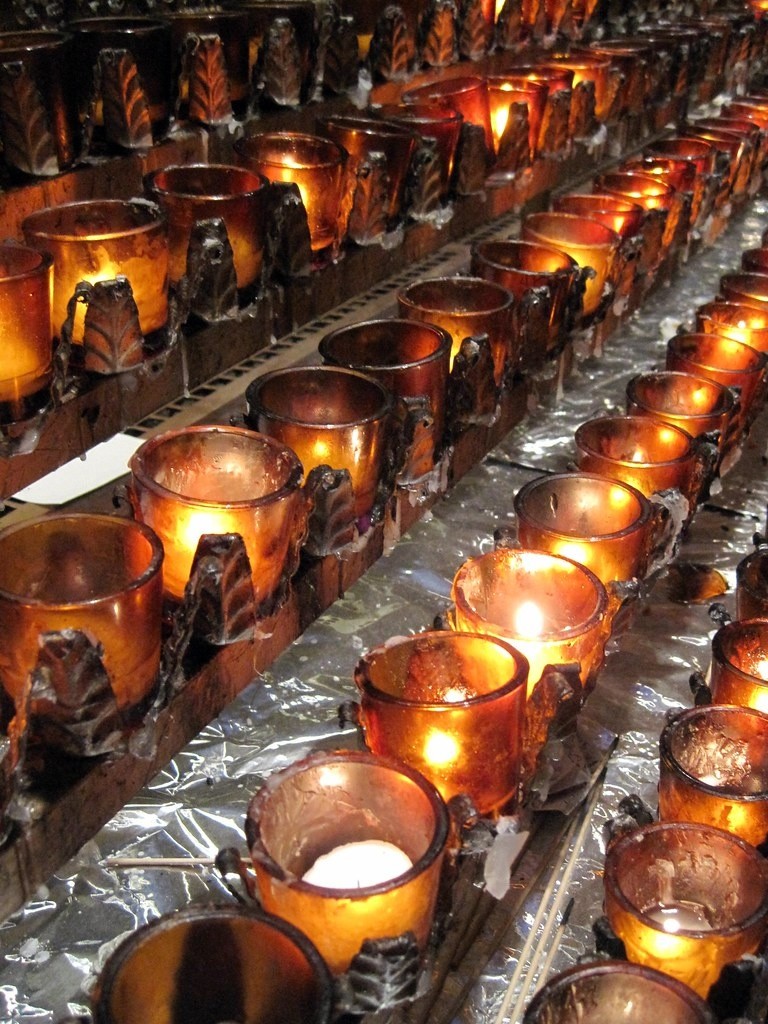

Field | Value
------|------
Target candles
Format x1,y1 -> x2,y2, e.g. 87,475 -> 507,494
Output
0,0 -> 768,1024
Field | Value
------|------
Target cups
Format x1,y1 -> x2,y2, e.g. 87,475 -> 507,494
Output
0,0 -> 768,1024
